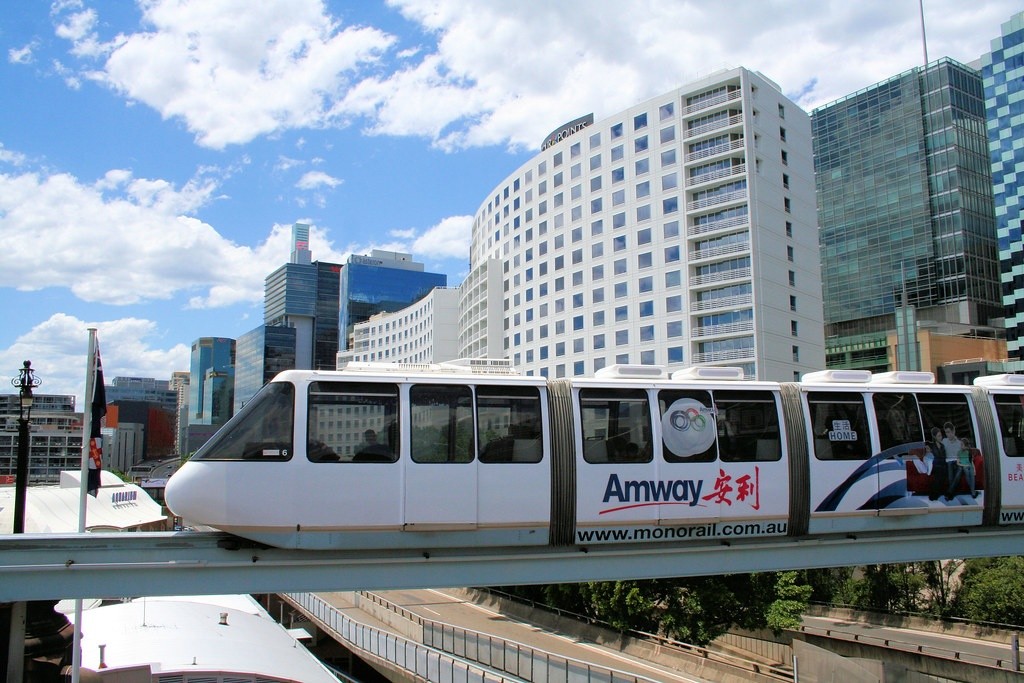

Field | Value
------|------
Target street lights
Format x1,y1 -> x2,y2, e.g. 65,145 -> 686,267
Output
11,361 -> 43,535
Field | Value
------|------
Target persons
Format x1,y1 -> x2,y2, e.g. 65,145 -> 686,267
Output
351,429 -> 396,462
898,421 -> 986,501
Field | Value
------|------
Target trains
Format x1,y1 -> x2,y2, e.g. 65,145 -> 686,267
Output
165,351 -> 1024,556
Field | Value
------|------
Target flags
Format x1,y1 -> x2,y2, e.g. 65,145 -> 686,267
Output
86,338 -> 107,499
